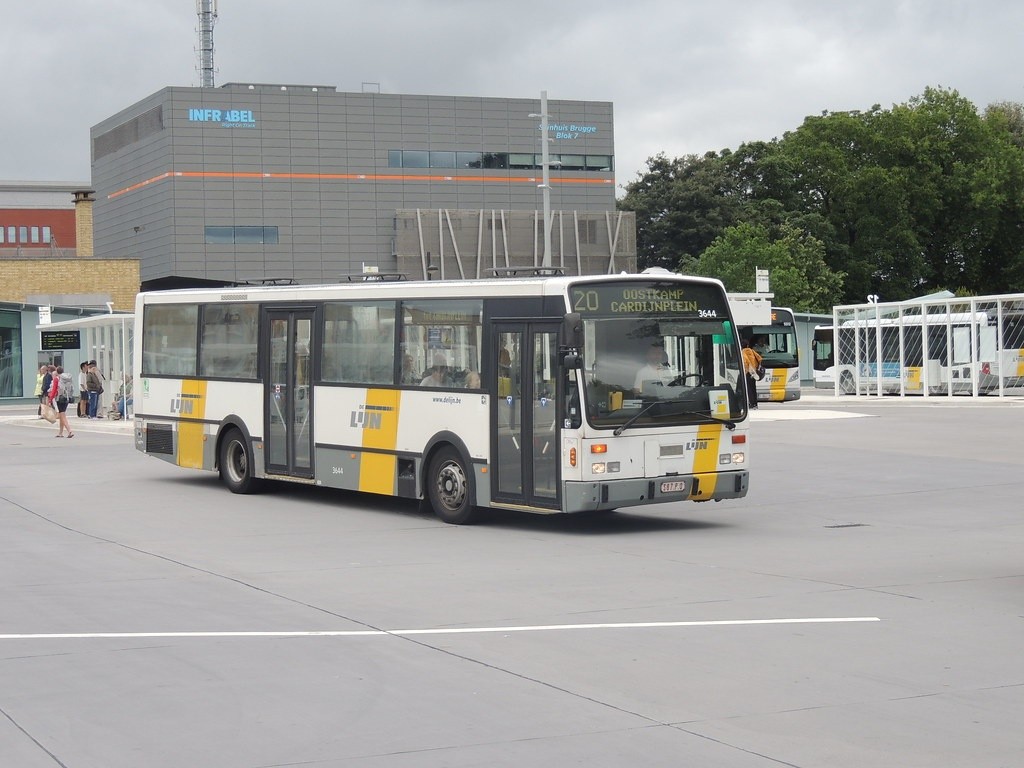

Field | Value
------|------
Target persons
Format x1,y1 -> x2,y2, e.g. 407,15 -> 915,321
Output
402,355 -> 417,383
114,375 -> 133,419
47,366 -> 74,438
420,355 -> 453,387
34,365 -> 60,419
741,340 -> 763,409
633,345 -> 674,394
79,359 -> 104,419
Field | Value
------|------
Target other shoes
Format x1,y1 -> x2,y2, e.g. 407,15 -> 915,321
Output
56,435 -> 65,437
67,432 -> 74,438
107,410 -> 122,420
749,403 -> 758,410
88,416 -> 99,421
80,414 -> 88,418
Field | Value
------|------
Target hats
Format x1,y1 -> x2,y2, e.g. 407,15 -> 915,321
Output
88,360 -> 96,366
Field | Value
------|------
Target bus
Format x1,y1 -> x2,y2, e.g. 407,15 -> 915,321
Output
811,304 -> 1024,396
132,267 -> 751,527
715,306 -> 801,403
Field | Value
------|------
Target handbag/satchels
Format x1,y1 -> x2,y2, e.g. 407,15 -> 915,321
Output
98,386 -> 104,394
41,399 -> 56,424
753,364 -> 765,381
